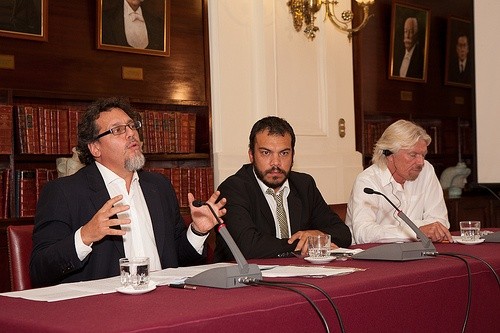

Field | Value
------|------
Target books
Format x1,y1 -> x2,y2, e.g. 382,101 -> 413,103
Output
0,104 -> 90,154
133,109 -> 197,153
0,166 -> 57,220
142,166 -> 214,207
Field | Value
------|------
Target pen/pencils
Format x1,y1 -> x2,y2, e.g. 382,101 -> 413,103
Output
330,252 -> 353,256
441,240 -> 453,243
167,284 -> 197,290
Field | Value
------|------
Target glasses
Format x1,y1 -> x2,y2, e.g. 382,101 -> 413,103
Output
95,121 -> 142,140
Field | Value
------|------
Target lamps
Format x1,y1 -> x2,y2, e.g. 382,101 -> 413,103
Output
287,0 -> 375,42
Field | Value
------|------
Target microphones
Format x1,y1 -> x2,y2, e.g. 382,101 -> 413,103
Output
183,199 -> 263,288
470,181 -> 500,243
352,187 -> 436,261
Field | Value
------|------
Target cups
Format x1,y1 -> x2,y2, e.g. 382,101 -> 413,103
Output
119,256 -> 150,291
460,221 -> 481,242
307,234 -> 331,260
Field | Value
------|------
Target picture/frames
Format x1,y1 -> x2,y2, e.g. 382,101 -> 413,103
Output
388,0 -> 432,83
95,0 -> 171,57
444,14 -> 475,88
0,0 -> 49,43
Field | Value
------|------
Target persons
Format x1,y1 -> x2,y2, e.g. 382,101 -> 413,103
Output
450,35 -> 473,83
344,120 -> 454,245
30,97 -> 227,286
211,116 -> 352,264
439,162 -> 471,198
396,17 -> 423,80
103,0 -> 164,50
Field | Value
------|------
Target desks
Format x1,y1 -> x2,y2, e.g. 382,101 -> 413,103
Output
0,224 -> 500,333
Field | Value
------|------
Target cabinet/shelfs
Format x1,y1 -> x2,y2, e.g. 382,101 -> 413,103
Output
362,113 -> 479,190
0,87 -> 215,292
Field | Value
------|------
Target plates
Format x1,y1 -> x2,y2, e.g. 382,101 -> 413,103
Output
117,282 -> 156,294
304,256 -> 336,264
457,238 -> 485,245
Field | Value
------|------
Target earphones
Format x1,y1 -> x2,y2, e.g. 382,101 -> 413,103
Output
382,150 -> 392,156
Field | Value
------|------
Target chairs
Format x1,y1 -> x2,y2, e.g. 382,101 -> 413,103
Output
6,224 -> 36,292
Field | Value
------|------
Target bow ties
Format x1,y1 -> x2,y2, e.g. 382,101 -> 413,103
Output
129,14 -> 144,22
404,53 -> 411,59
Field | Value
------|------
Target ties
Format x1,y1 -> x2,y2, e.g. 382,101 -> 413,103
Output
461,62 -> 464,72
266,187 -> 289,239
